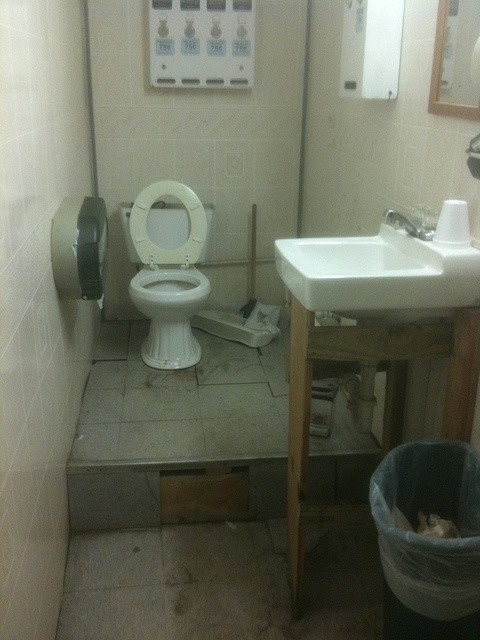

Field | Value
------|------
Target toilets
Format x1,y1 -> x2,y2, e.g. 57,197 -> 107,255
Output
117,179 -> 214,370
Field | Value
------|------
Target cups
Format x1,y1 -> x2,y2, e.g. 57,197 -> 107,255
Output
432,199 -> 471,249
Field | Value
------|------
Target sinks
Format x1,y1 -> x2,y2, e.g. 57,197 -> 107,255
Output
274,224 -> 480,312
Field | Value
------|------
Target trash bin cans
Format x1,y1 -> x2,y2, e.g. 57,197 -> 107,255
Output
368,440 -> 480,640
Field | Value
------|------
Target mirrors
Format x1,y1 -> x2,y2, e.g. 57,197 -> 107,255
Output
428,0 -> 480,122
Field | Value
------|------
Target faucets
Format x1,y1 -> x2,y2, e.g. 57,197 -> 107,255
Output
383,208 -> 424,239
